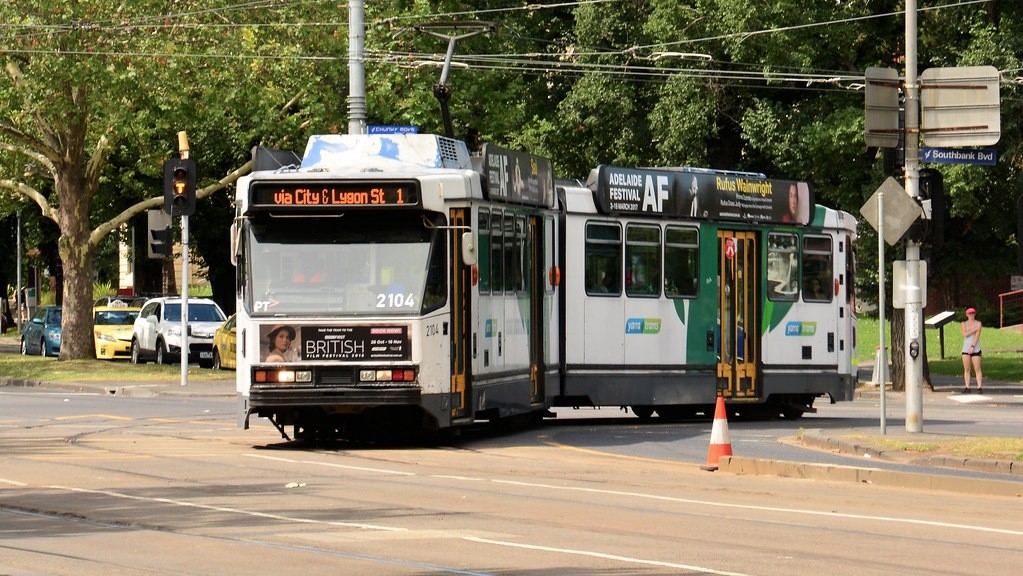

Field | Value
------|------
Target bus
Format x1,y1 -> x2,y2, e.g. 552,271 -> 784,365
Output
226,122 -> 863,447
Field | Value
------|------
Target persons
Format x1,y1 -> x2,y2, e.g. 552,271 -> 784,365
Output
782,183 -> 799,223
586,268 -> 609,293
688,175 -> 699,216
960,307 -> 984,395
803,276 -> 828,300
265,324 -> 297,362
513,154 -> 524,198
15,288 -> 27,322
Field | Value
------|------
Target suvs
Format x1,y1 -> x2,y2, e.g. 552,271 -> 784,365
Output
128,295 -> 229,369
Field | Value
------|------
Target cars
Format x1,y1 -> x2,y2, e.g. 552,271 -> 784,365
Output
130,296 -> 155,309
92,296 -> 135,307
20,306 -> 63,358
211,313 -> 238,370
91,299 -> 143,361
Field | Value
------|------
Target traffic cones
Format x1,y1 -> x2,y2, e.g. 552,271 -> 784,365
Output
700,395 -> 734,470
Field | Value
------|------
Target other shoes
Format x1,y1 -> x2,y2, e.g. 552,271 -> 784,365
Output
962,388 -> 972,394
977,388 -> 983,394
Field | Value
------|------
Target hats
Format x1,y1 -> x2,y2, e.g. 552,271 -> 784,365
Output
266,325 -> 297,342
966,309 -> 976,314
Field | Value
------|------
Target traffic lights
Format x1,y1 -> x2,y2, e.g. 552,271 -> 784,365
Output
163,158 -> 196,217
150,228 -> 174,256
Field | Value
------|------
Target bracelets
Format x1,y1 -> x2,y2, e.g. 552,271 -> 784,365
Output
971,345 -> 974,348
